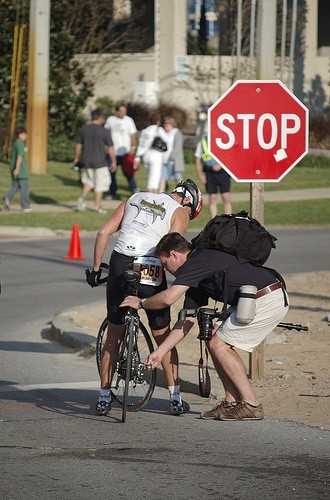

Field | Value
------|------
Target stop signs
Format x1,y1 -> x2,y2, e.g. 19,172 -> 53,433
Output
207,79 -> 309,182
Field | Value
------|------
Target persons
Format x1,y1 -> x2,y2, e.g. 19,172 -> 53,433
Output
89,179 -> 204,421
73,104 -> 187,217
194,120 -> 233,222
118,232 -> 290,420
4,127 -> 34,212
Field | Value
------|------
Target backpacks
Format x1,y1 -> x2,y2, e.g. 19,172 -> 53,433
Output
187,210 -> 278,289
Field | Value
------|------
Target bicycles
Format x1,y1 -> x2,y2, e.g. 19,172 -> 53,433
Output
84,262 -> 157,423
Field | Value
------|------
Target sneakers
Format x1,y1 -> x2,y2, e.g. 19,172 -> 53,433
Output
200,398 -> 238,419
218,401 -> 264,420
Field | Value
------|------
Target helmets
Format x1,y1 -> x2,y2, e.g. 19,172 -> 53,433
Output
172,178 -> 203,219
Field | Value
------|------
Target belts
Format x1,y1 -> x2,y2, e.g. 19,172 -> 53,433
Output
256,282 -> 282,299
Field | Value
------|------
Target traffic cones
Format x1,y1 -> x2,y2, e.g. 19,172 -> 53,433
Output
63,223 -> 86,260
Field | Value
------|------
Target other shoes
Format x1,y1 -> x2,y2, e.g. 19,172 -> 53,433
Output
96,397 -> 115,415
169,399 -> 191,413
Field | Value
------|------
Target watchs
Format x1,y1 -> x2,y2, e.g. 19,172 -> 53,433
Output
140,298 -> 147,309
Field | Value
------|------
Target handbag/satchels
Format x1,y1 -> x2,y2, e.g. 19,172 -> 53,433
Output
151,136 -> 168,152
